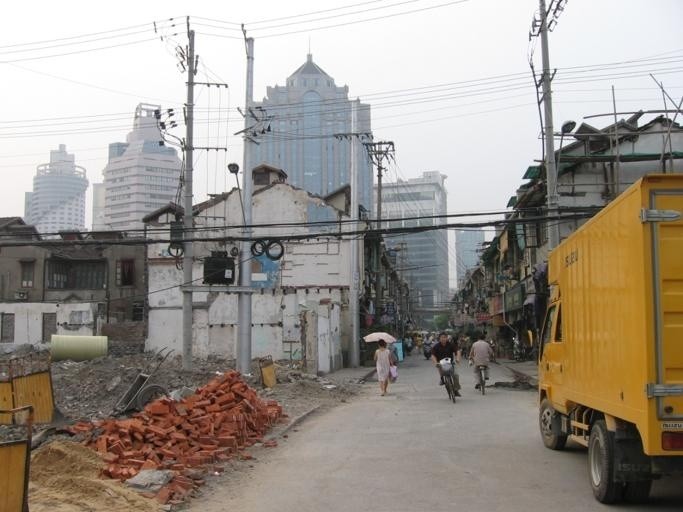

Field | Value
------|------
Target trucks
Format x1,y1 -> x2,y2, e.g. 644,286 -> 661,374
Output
535,171 -> 683,507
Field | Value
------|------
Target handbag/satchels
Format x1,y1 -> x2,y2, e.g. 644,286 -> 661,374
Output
388,366 -> 396,382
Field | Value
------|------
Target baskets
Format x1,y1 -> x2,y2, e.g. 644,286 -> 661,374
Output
439,363 -> 455,377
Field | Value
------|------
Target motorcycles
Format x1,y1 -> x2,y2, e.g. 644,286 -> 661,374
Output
422,341 -> 432,359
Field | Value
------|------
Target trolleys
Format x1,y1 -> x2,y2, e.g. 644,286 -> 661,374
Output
107,345 -> 175,417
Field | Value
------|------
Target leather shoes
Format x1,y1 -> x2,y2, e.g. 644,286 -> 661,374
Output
439,380 -> 443,385
454,391 -> 461,397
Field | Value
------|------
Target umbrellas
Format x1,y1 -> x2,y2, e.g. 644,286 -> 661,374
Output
362,330 -> 396,343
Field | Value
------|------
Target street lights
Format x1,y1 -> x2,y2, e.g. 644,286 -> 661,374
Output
547,119 -> 577,253
227,161 -> 252,381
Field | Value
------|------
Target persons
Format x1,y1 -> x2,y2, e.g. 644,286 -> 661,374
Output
373,339 -> 394,396
405,333 -> 498,359
468,334 -> 493,390
430,332 -> 462,397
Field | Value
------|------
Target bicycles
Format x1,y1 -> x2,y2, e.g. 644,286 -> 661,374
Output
468,357 -> 489,395
434,360 -> 459,403
